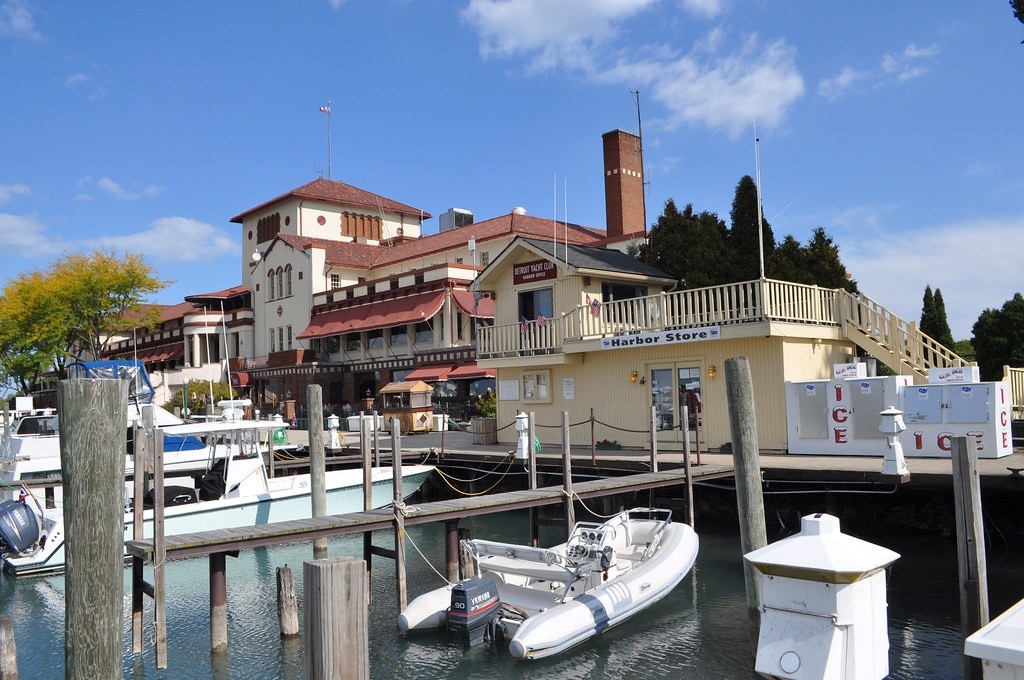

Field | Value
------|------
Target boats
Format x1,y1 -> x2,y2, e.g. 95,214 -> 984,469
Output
0,361 -> 291,510
1,399 -> 434,577
397,507 -> 700,662
